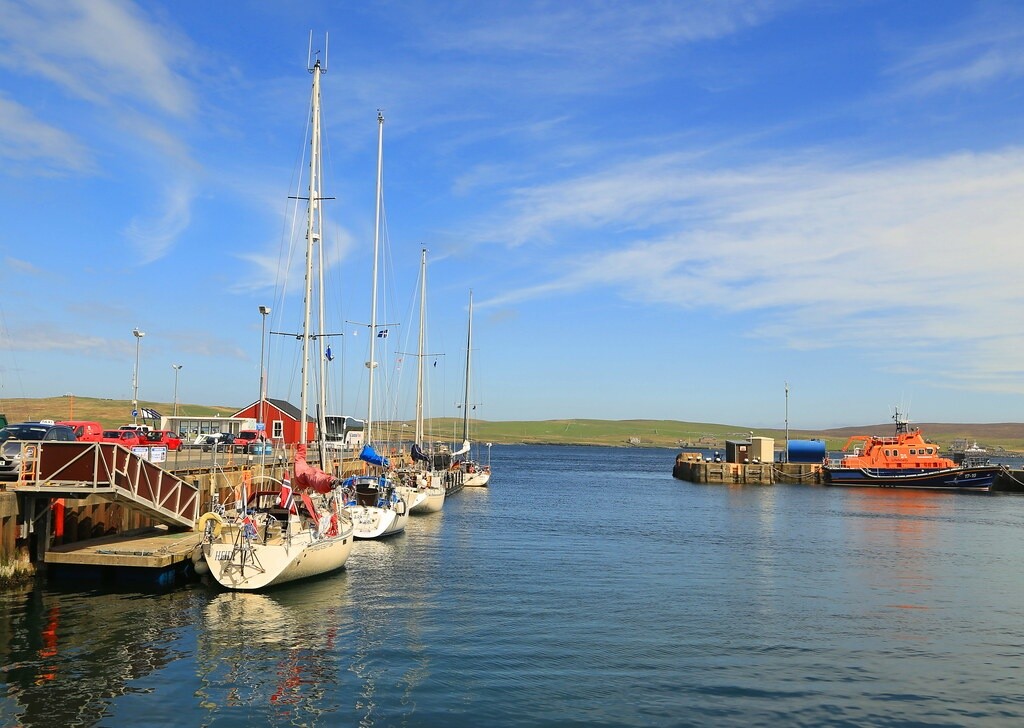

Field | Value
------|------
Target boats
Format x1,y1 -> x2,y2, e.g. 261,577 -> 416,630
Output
824,406 -> 1024,497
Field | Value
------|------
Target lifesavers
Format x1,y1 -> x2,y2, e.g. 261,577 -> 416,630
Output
198,512 -> 223,538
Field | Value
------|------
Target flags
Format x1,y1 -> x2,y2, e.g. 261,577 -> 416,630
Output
358,442 -> 390,469
378,328 -> 389,338
279,466 -> 299,515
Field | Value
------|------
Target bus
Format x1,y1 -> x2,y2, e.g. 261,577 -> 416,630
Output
314,415 -> 366,451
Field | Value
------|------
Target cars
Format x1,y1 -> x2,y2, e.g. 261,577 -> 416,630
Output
232,429 -> 268,452
202,432 -> 236,453
144,429 -> 183,451
0,420 -> 151,480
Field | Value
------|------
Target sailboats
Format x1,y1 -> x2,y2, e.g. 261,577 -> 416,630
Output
194,28 -> 494,589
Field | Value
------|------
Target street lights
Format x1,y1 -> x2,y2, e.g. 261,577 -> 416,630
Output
256,303 -> 269,430
172,364 -> 182,416
131,328 -> 145,425
